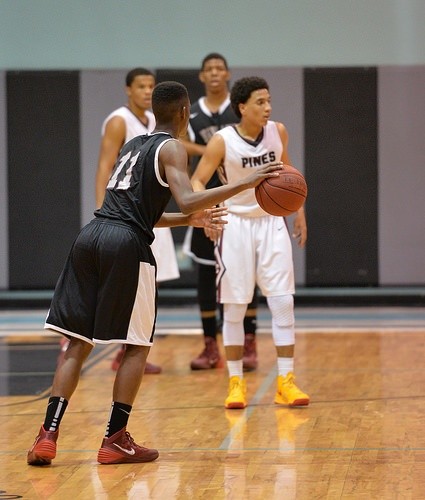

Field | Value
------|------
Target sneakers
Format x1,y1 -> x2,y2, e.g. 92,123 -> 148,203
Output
96,426 -> 159,464
112,347 -> 161,374
224,375 -> 247,407
191,349 -> 220,368
274,373 -> 309,407
28,425 -> 60,465
241,348 -> 258,372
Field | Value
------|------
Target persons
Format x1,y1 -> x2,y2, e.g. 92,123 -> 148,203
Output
184,51 -> 261,370
25,80 -> 284,467
97,66 -> 179,376
191,76 -> 312,408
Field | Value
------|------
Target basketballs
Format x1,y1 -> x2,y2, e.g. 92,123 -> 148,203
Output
254,163 -> 308,216
